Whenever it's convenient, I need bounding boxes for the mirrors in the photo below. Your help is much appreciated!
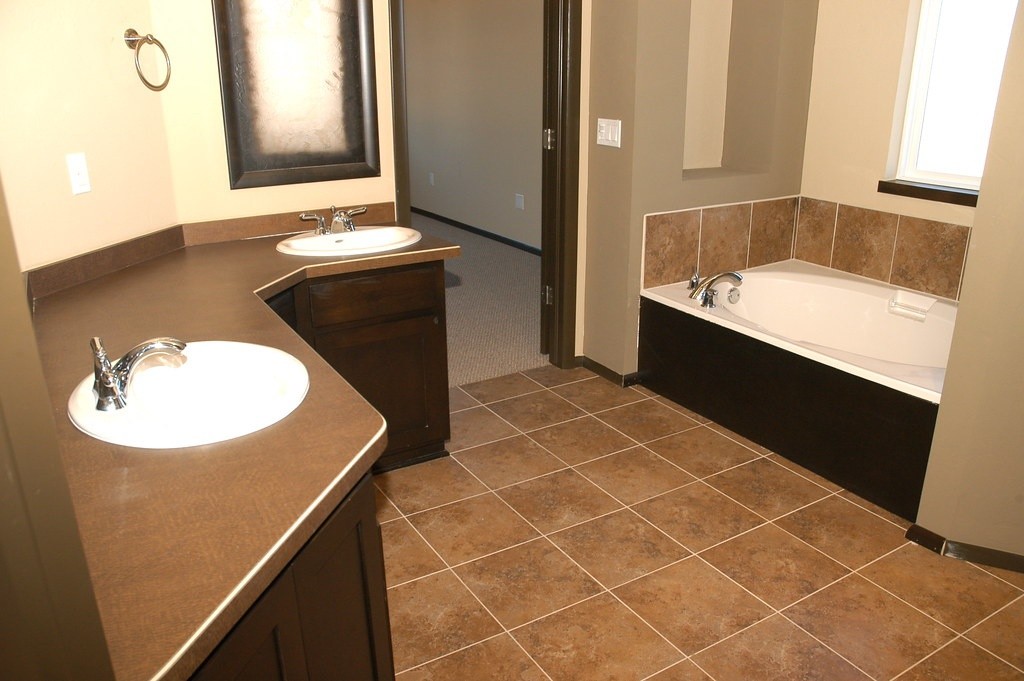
[211,0,382,192]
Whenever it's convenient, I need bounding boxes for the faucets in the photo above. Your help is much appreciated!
[112,334,187,385]
[689,268,743,300]
[330,210,355,233]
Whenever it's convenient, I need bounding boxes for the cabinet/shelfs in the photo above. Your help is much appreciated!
[177,462,397,681]
[267,259,450,476]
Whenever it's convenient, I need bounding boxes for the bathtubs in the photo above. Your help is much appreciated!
[636,257,959,530]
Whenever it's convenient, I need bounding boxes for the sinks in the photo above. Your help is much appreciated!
[275,222,423,257]
[67,335,312,451]
